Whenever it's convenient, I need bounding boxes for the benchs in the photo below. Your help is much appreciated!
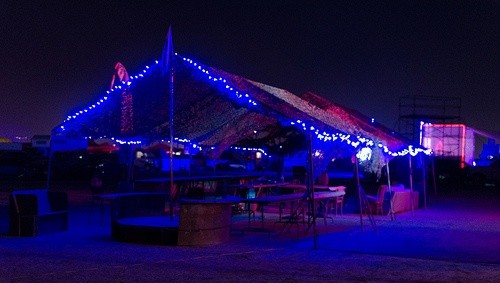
[94,171,421,240]
[9,188,70,240]
